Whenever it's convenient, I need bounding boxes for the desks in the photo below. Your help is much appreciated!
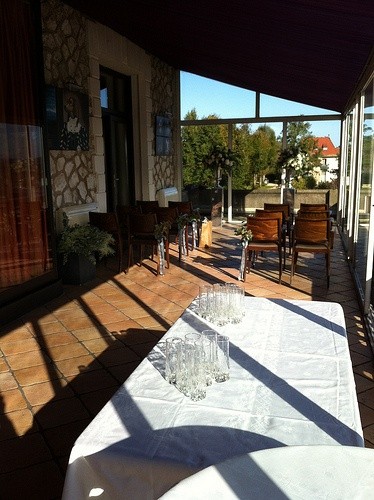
[62,293,365,500]
[158,445,374,500]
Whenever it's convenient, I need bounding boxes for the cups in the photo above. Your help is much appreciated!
[165,330,231,402]
[199,282,246,326]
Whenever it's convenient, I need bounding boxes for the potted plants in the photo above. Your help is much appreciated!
[54,213,116,285]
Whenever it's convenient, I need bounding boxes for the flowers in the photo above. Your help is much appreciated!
[278,145,307,171]
[202,145,234,171]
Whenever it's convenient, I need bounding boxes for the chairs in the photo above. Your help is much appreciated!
[241,203,334,289]
[89,200,202,277]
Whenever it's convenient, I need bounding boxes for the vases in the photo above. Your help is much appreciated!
[214,163,222,188]
[285,167,293,188]
